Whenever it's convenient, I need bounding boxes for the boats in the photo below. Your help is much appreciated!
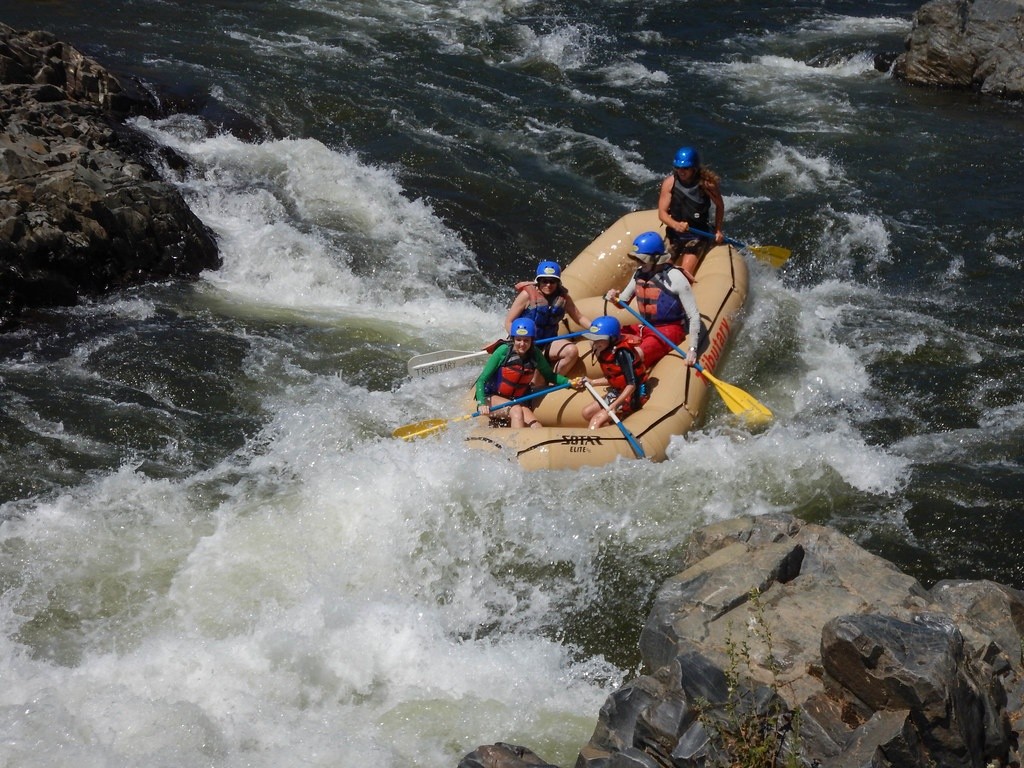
[460,209,751,469]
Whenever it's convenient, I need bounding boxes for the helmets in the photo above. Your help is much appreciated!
[633,231,665,255]
[535,261,561,278]
[672,146,698,167]
[590,315,620,337]
[510,317,536,336]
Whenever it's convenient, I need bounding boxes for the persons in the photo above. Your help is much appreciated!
[580,316,649,430]
[604,231,701,369]
[504,261,594,388]
[658,147,725,283]
[468,318,581,429]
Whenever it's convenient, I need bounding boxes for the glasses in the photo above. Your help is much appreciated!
[537,278,557,284]
[674,167,692,170]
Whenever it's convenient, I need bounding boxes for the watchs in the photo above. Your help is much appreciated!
[688,346,696,352]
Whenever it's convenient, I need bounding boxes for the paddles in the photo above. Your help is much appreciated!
[686,225,792,269]
[405,328,590,378]
[389,381,576,441]
[583,380,648,461]
[611,294,775,426]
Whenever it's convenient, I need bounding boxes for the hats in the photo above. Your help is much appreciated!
[533,275,562,288]
[581,333,616,342]
[627,252,671,265]
